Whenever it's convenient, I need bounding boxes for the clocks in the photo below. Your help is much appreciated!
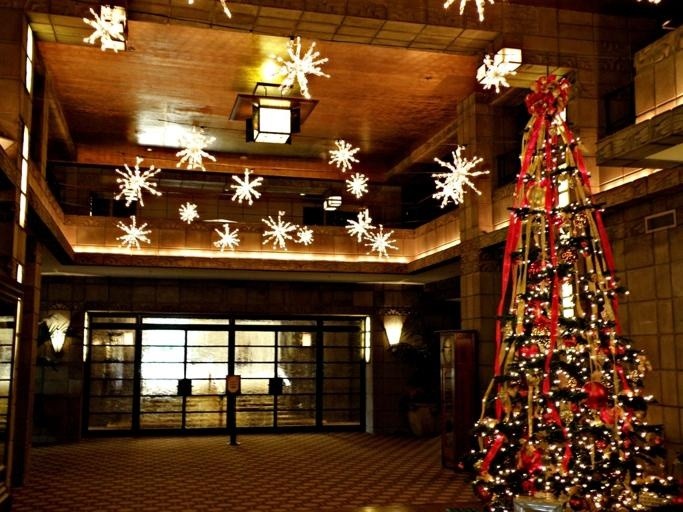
[433,330,476,470]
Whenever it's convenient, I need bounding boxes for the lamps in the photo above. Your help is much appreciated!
[477,48,522,84]
[322,189,342,212]
[38,304,80,353]
[378,307,412,352]
[228,94,320,146]
